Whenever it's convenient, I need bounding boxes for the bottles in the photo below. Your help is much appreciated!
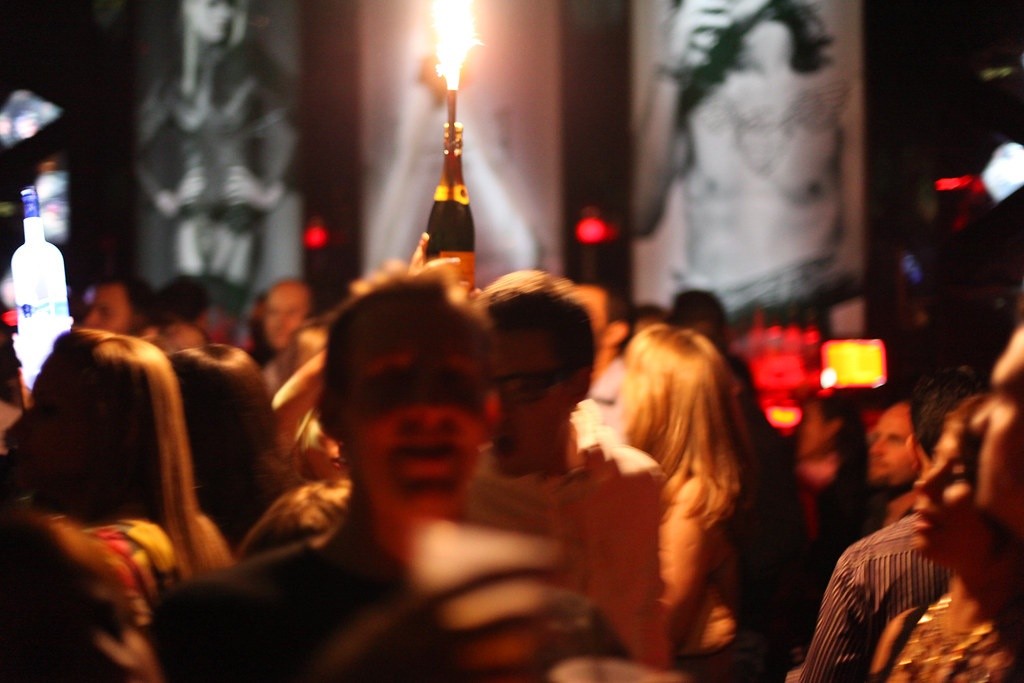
[427,121,476,290]
[15,187,71,409]
[747,303,826,388]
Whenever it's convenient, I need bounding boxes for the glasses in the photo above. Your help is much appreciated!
[336,366,488,415]
[484,364,586,405]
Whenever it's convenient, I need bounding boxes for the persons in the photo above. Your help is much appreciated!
[0,234,1024,683]
[134,0,298,283]
[633,0,849,307]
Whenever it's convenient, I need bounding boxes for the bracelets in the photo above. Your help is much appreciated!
[651,60,695,88]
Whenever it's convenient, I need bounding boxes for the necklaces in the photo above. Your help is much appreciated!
[897,594,1004,666]
